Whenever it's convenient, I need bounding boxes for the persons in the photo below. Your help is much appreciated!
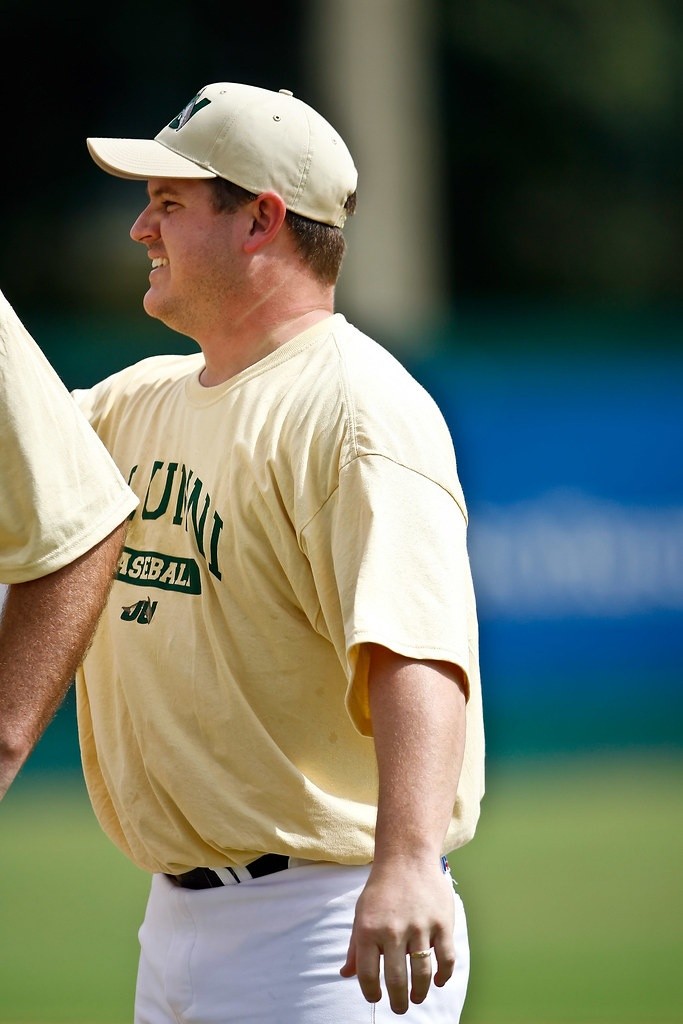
[0,291,141,803]
[69,82,486,1023]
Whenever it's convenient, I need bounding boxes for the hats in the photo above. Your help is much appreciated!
[86,82,359,229]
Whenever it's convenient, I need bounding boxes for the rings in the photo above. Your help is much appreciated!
[410,949,431,959]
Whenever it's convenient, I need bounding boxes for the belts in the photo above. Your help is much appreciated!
[164,852,289,891]
